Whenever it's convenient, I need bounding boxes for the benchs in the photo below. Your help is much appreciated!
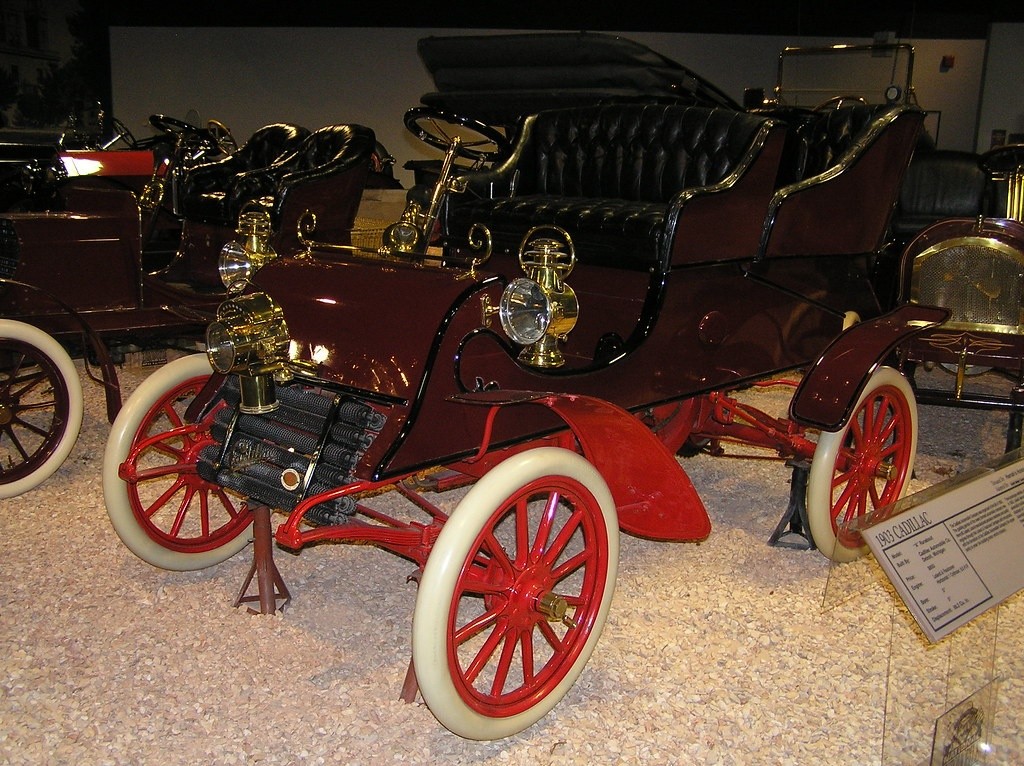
[445,103,786,270]
[746,102,928,258]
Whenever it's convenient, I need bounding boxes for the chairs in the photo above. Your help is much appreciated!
[183,124,312,226]
[895,215,1024,454]
[228,123,377,232]
